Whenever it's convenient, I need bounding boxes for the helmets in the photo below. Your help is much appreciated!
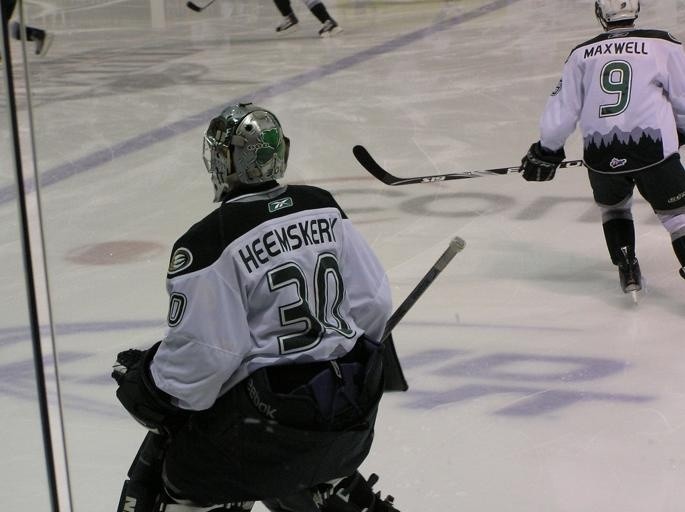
[202,103,290,202]
[595,0,640,23]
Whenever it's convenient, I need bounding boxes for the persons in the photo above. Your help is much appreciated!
[522,3,685,301]
[272,1,344,39]
[9,22,53,58]
[106,102,410,511]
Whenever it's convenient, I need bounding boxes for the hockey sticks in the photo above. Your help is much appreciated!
[187,1,215,12]
[353,145,586,186]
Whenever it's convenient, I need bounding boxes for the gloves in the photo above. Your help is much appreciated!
[518,141,565,181]
[111,341,190,436]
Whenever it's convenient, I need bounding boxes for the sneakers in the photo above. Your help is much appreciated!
[31,28,44,55]
[318,18,338,33]
[619,257,641,292]
[276,13,298,32]
